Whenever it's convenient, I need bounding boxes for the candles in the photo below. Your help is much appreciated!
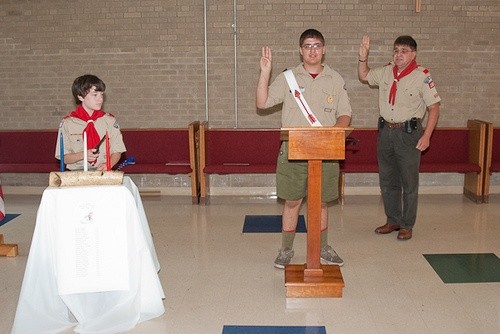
[60,130,65,172]
[83,130,88,172]
[105,130,111,171]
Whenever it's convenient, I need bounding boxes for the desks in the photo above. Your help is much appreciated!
[12,176,166,333]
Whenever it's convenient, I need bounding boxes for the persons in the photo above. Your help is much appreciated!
[55,73,127,172]
[358,35,441,239]
[256,29,352,269]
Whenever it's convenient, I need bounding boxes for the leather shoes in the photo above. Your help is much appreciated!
[376,224,400,233]
[397,229,412,239]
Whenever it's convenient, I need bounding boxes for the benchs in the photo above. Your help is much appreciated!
[0,121,500,204]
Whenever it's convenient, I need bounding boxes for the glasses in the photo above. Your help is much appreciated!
[392,50,412,54]
[302,43,322,50]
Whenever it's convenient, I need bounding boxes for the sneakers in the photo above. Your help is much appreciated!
[318,245,344,266]
[274,249,293,269]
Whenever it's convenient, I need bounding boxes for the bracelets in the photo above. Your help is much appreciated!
[359,59,367,62]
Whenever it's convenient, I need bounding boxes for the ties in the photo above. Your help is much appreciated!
[388,60,416,105]
[70,104,107,164]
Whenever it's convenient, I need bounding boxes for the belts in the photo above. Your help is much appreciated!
[386,121,407,128]
